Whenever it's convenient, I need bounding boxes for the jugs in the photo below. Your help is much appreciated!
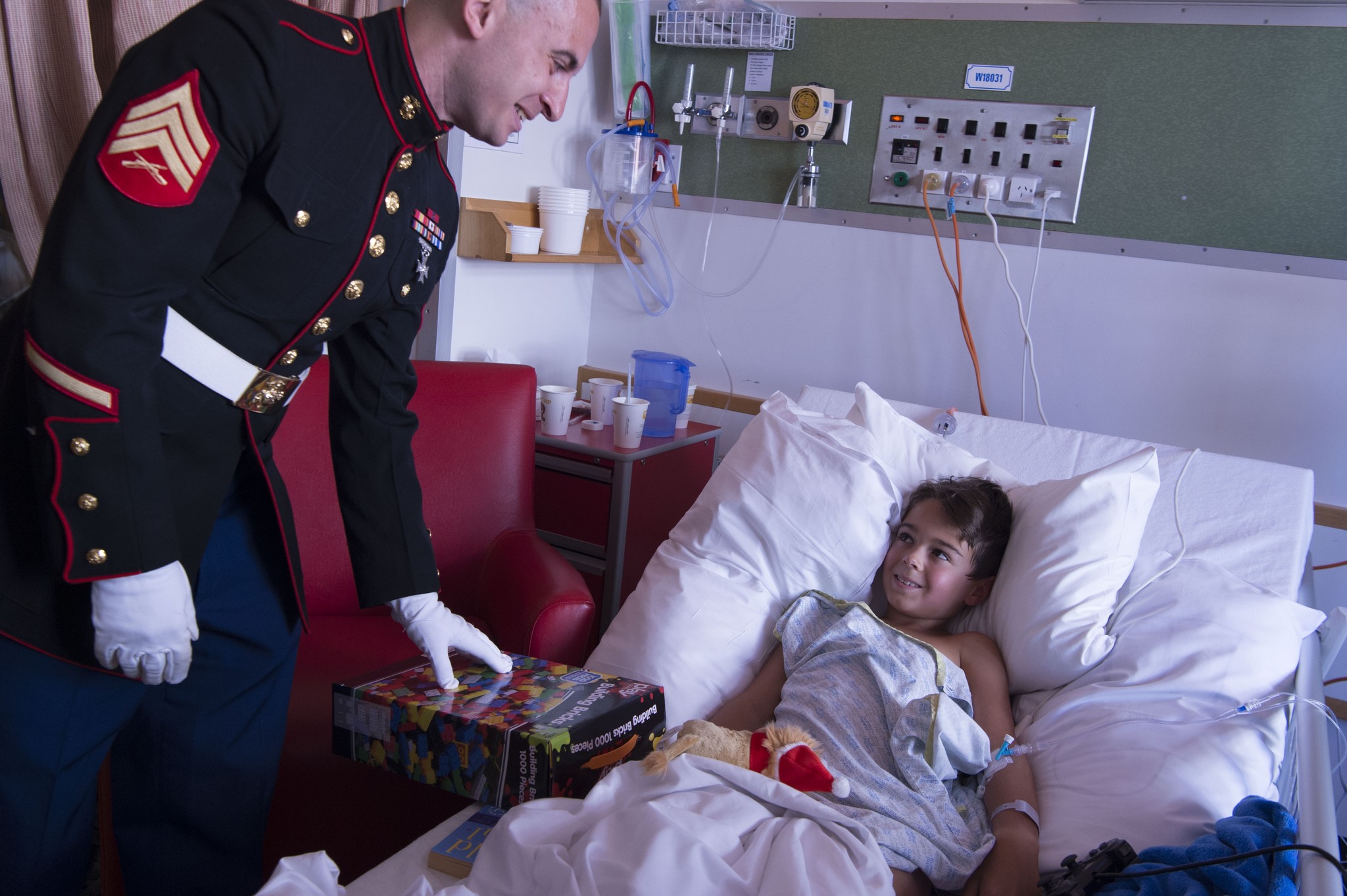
[630,349,697,437]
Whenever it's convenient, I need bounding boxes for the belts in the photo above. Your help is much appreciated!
[158,306,312,417]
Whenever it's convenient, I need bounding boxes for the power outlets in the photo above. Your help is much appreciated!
[654,145,682,193]
[920,168,948,195]
[977,174,1005,201]
[1007,177,1037,203]
[949,170,977,198]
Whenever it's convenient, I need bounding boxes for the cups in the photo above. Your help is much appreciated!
[668,381,697,429]
[611,397,650,448]
[508,226,544,254]
[538,186,590,254]
[588,378,624,425]
[539,386,577,436]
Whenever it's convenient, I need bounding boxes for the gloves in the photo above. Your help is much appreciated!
[384,592,513,689]
[90,561,199,686]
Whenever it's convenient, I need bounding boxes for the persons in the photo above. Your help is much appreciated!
[0,0,602,896]
[712,474,1042,896]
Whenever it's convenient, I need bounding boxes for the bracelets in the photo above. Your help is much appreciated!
[989,799,1040,831]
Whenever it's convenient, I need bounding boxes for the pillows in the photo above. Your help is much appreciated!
[844,381,1160,689]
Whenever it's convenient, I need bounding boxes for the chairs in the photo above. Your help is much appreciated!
[258,356,596,886]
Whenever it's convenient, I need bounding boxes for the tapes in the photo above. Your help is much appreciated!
[581,419,604,430]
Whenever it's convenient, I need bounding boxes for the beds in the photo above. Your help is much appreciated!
[254,381,1344,896]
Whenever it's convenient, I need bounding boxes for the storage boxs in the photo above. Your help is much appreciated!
[331,646,667,809]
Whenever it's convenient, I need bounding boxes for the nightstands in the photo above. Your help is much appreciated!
[534,408,721,667]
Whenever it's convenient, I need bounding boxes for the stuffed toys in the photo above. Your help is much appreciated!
[642,718,852,799]
